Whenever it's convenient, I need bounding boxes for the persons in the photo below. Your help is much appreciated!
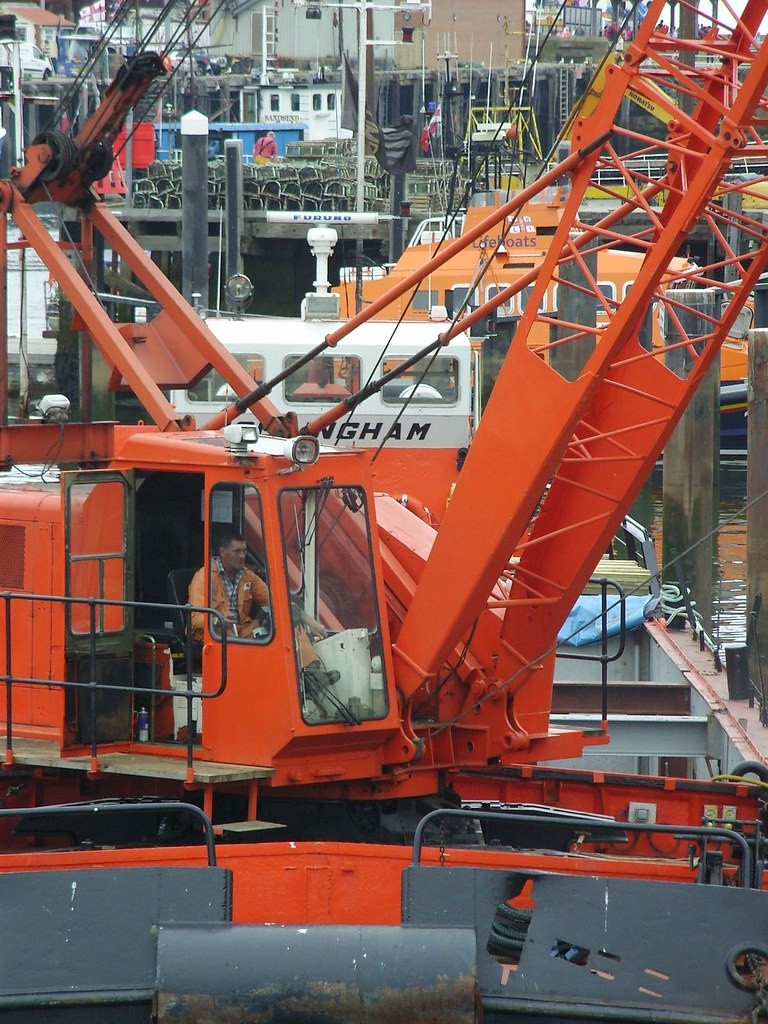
[185,528,341,696]
[43,40,51,61]
[314,364,329,388]
[575,19,713,43]
[252,131,279,167]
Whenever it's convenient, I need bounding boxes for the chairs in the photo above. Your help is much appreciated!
[166,567,204,657]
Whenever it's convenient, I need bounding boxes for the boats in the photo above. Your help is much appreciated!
[168,191,756,476]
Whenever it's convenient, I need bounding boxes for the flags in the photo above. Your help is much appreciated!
[421,104,441,153]
[154,100,162,124]
[159,52,172,75]
[79,0,106,23]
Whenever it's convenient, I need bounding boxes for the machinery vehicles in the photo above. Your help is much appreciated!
[0,0,768,1024]
